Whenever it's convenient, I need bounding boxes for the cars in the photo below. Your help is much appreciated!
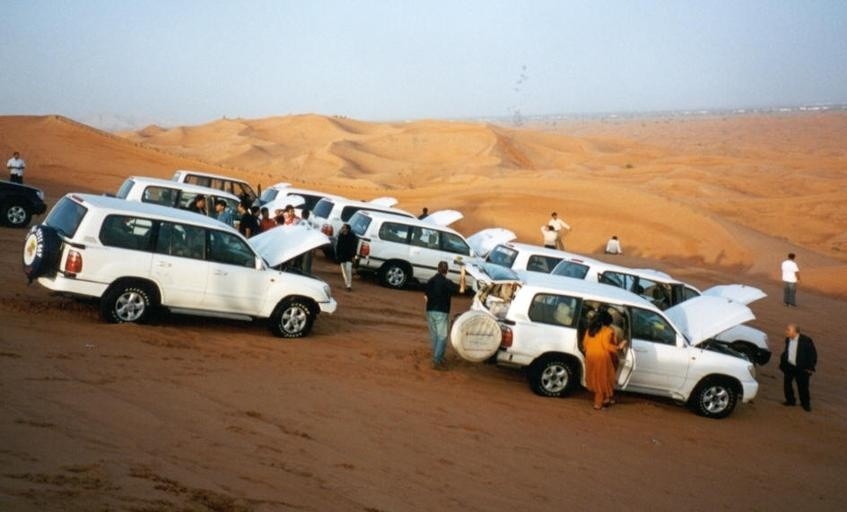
[0,176,48,227]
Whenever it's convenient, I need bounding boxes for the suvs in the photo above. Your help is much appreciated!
[465,235,671,308]
[18,190,341,341]
[447,257,764,422]
[547,254,772,369]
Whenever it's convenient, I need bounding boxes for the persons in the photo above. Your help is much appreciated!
[5,150,26,183]
[580,310,629,411]
[539,224,558,248]
[779,253,800,306]
[335,224,357,292]
[190,194,313,276]
[423,259,465,370]
[547,211,570,249]
[604,235,622,255]
[776,321,816,413]
[416,207,428,220]
